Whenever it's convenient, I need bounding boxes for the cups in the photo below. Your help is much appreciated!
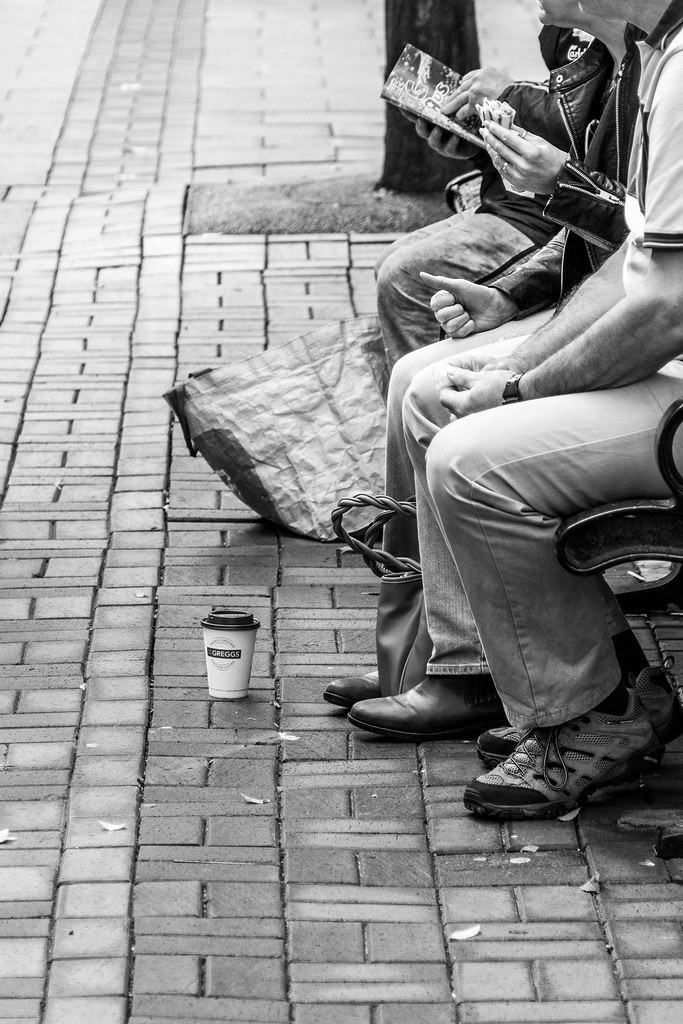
[201,610,261,698]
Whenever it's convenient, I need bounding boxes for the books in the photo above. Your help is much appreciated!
[379,42,486,150]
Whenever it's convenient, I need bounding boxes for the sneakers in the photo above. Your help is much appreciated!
[476,653,683,768]
[463,673,666,820]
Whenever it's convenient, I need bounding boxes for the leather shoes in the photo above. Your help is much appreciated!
[347,674,508,742]
[323,670,382,707]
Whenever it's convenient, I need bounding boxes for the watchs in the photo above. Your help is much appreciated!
[502,372,527,404]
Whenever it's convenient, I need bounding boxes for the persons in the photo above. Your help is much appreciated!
[323,0,683,821]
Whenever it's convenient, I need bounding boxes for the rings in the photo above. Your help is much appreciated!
[503,161,509,175]
[521,130,528,139]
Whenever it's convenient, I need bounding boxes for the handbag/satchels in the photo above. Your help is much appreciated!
[161,313,391,543]
[330,492,435,699]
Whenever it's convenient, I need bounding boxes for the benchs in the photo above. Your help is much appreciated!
[443,166,683,612]
